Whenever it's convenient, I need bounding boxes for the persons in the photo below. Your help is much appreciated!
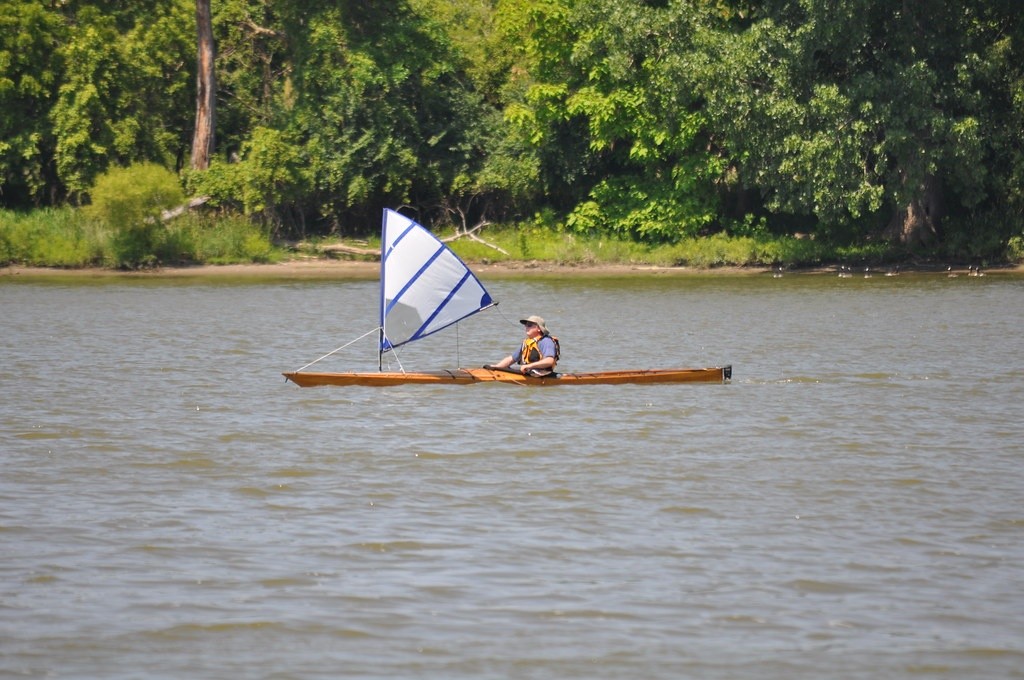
[491,316,557,376]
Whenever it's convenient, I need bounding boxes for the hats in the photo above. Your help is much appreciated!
[519,316,550,336]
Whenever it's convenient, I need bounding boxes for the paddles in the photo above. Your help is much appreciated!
[482,365,558,378]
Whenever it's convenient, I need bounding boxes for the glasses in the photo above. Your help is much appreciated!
[525,323,537,327]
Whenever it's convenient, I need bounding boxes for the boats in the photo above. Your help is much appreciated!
[285,365,732,389]
